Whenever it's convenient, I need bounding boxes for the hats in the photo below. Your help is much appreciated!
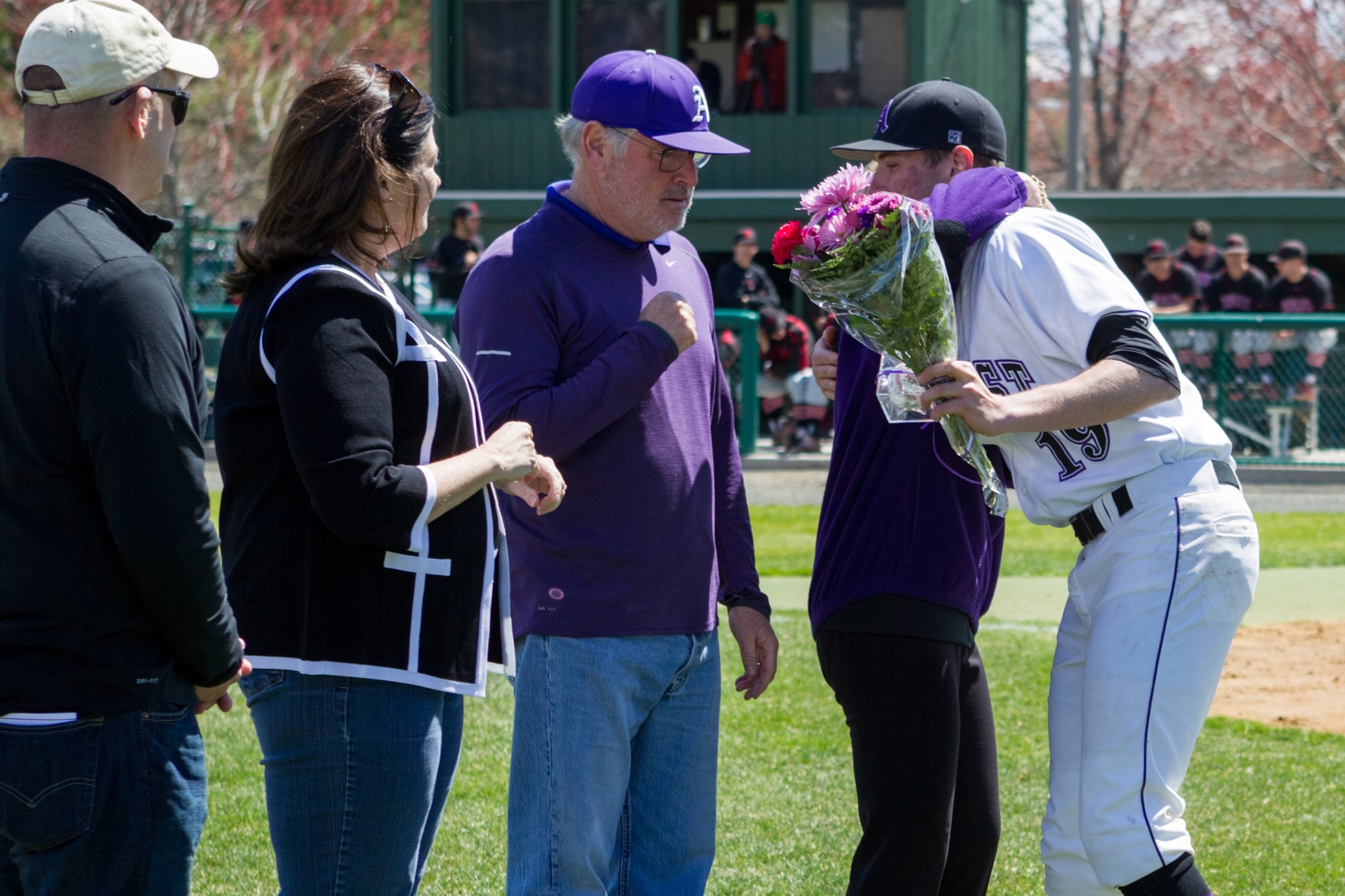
[1220,232,1249,255]
[759,308,787,336]
[450,200,490,218]
[1266,238,1308,263]
[571,49,751,155]
[1188,218,1214,242]
[1144,237,1169,261]
[829,77,1007,163]
[15,0,219,108]
[733,228,759,247]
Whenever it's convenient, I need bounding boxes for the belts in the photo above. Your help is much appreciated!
[1069,460,1239,546]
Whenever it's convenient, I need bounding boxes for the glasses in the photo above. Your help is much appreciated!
[110,85,190,126]
[376,60,423,162]
[607,126,712,172]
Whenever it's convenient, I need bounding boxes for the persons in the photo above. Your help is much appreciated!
[208,58,571,896]
[458,48,782,896]
[0,0,249,896]
[797,75,1260,896]
[194,10,1338,462]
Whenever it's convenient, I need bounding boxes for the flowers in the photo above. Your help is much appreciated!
[771,163,1013,522]
